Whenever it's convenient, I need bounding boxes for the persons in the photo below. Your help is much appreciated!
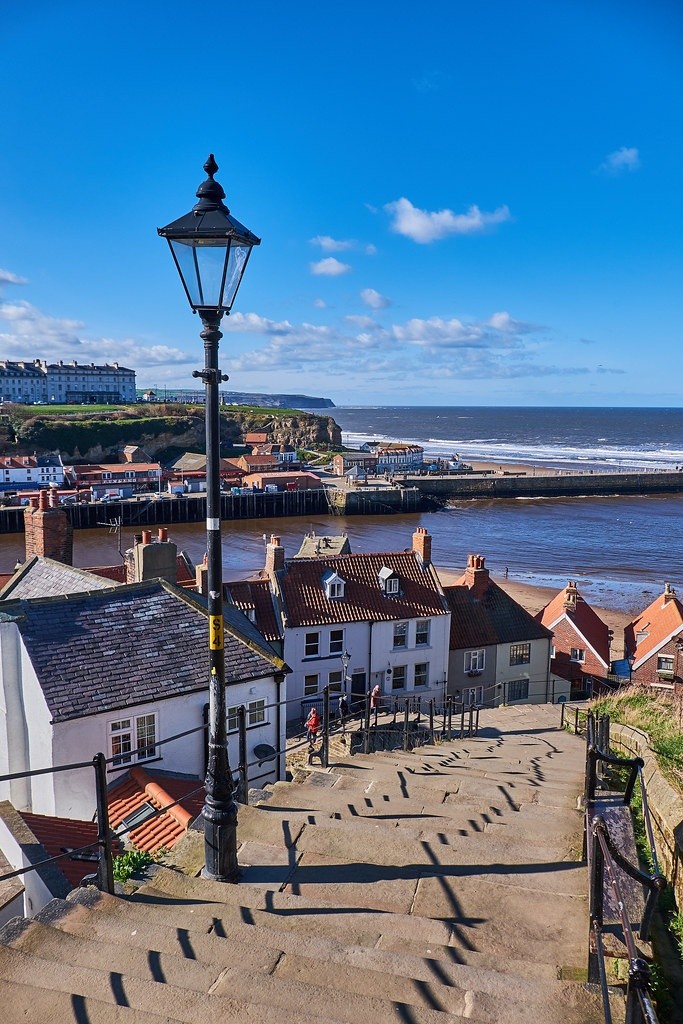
[370,685,380,713]
[304,708,319,743]
[338,696,348,725]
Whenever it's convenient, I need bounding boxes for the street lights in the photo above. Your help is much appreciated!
[156,149,265,891]
